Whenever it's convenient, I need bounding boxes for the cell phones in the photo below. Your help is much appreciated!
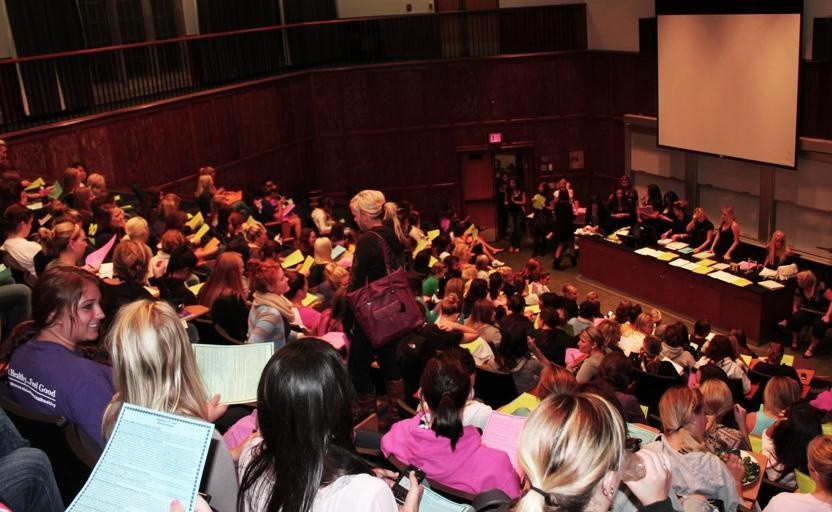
[391,464,426,505]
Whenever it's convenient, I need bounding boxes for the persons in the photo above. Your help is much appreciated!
[1,158,832,511]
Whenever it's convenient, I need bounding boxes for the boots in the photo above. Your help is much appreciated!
[350,392,378,426]
[377,377,407,433]
[570,254,577,266]
[552,257,566,272]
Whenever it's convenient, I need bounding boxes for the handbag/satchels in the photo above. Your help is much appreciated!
[343,266,427,351]
